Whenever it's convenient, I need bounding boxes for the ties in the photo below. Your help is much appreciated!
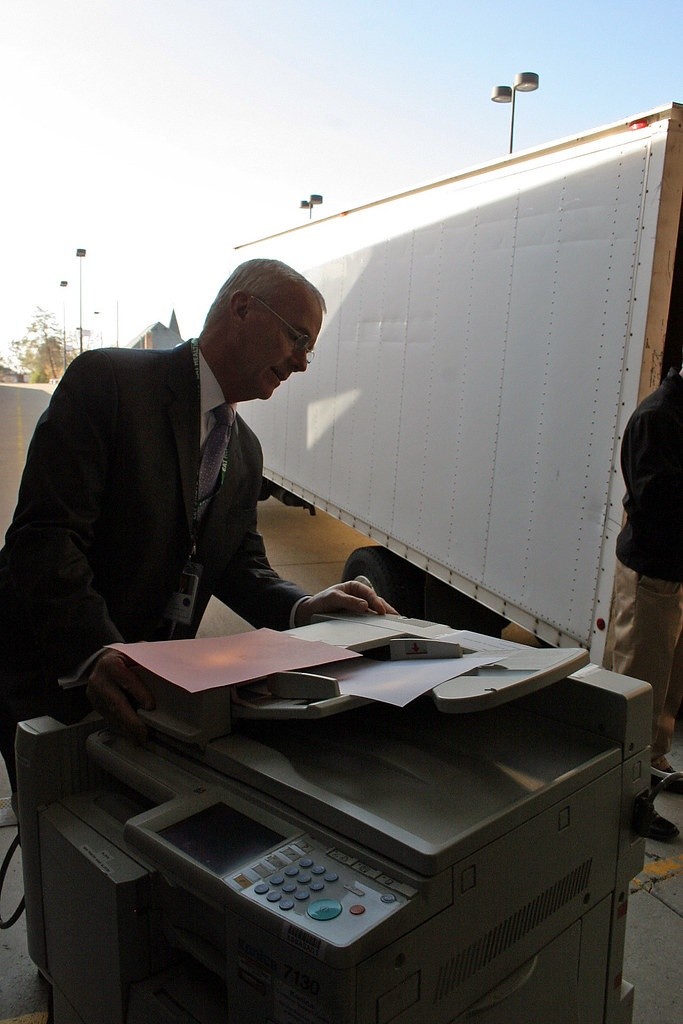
[196,403,235,538]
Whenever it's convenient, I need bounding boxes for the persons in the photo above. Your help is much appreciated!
[611,367,683,840]
[0,260,397,834]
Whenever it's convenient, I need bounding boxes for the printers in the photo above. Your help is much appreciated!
[14,604,678,1024]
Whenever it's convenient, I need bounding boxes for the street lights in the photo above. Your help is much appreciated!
[490,70,539,157]
[94,310,104,348]
[76,246,87,354]
[299,193,324,221]
[58,279,69,374]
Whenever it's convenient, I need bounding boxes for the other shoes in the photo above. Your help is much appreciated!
[651,766,683,794]
[645,809,679,842]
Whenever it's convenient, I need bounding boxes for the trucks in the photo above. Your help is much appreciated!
[198,105,682,706]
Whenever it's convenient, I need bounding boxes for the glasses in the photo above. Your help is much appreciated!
[251,294,315,364]
